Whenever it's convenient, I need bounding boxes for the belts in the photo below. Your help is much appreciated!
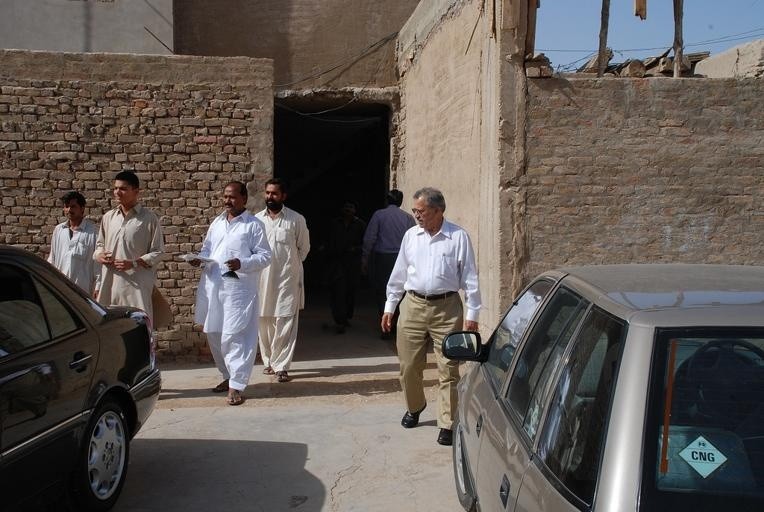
[406,290,456,302]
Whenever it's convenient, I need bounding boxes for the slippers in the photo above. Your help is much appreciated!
[211,379,229,393]
[262,366,274,375]
[225,388,243,406]
[276,370,289,382]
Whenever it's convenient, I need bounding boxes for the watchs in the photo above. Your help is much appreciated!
[131,259,138,271]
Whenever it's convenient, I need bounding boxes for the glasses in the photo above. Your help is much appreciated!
[410,208,425,215]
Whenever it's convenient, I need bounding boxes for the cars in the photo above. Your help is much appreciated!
[441,265,763,511]
[1,237,160,512]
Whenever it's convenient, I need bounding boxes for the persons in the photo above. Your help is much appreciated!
[93,171,164,372]
[187,182,272,405]
[362,189,415,339]
[46,191,103,301]
[316,200,366,334]
[255,179,311,381]
[381,186,481,446]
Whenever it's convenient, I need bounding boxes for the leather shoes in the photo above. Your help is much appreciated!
[436,427,453,446]
[400,402,427,428]
